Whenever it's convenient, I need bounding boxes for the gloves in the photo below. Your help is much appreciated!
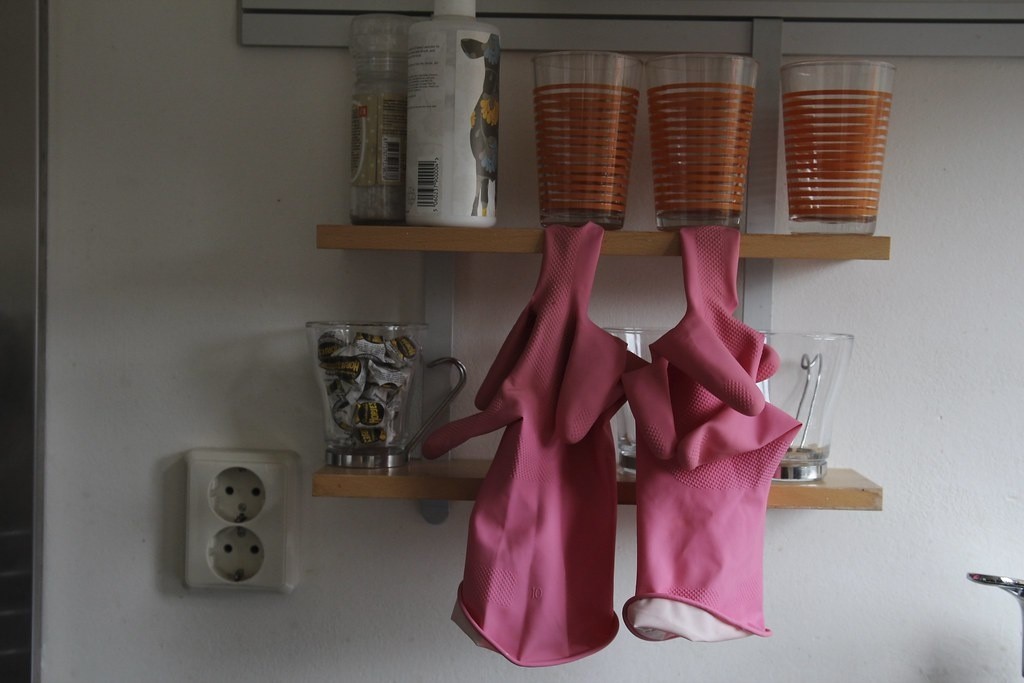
[421,220,803,666]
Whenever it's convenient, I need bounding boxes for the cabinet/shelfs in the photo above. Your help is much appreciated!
[308,222,894,514]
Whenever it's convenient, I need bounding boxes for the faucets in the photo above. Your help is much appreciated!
[967,573,1024,677]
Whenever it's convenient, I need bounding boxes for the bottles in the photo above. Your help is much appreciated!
[406,0,501,228]
[342,14,417,225]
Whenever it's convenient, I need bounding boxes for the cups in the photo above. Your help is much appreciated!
[530,51,644,230]
[645,53,760,232]
[780,60,897,236]
[304,320,469,469]
[759,331,856,483]
[603,327,672,478]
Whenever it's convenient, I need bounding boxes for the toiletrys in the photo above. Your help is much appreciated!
[407,1,500,225]
[349,17,415,221]
